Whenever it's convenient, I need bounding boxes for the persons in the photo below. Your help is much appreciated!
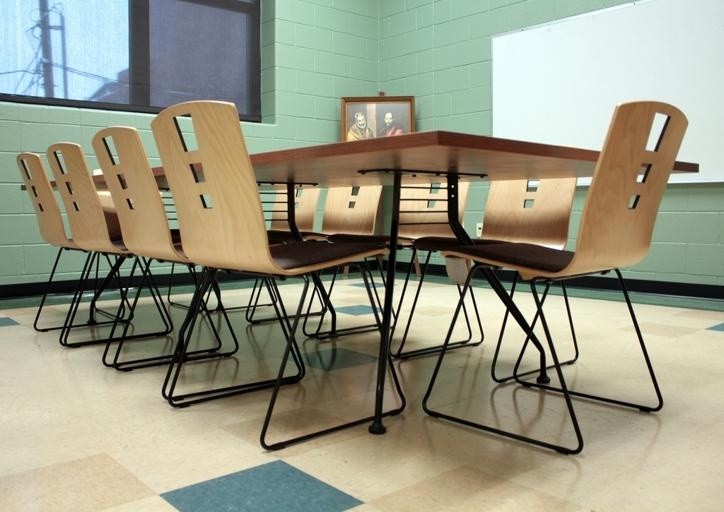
[347,111,373,142]
[375,112,404,138]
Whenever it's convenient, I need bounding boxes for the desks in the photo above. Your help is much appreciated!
[20,130,700,435]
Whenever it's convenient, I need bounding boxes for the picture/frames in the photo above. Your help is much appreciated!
[339,96,416,144]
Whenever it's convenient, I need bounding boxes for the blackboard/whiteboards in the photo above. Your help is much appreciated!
[492,1,724,182]
